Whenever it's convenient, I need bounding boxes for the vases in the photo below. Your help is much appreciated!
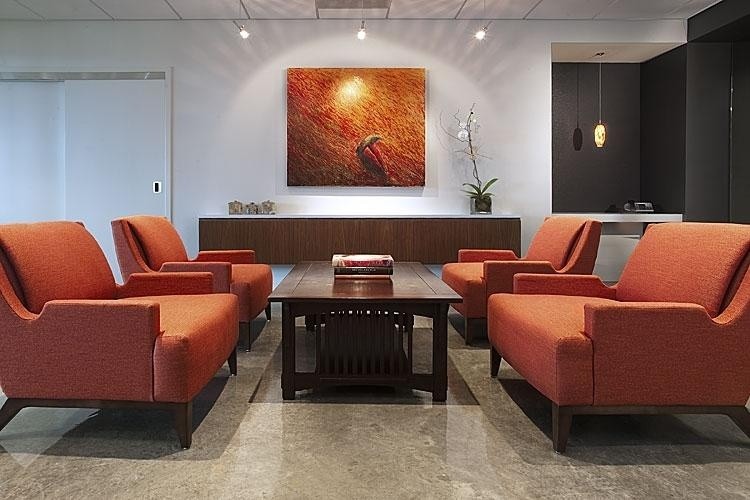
[470,199,493,215]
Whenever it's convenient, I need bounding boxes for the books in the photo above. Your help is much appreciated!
[333,274,391,279]
[331,252,395,268]
[333,267,394,276]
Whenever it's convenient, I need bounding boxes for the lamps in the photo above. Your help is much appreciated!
[593,51,607,148]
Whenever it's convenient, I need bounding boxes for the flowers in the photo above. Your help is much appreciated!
[439,97,500,199]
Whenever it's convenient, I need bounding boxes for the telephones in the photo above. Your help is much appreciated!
[624,200,654,213]
